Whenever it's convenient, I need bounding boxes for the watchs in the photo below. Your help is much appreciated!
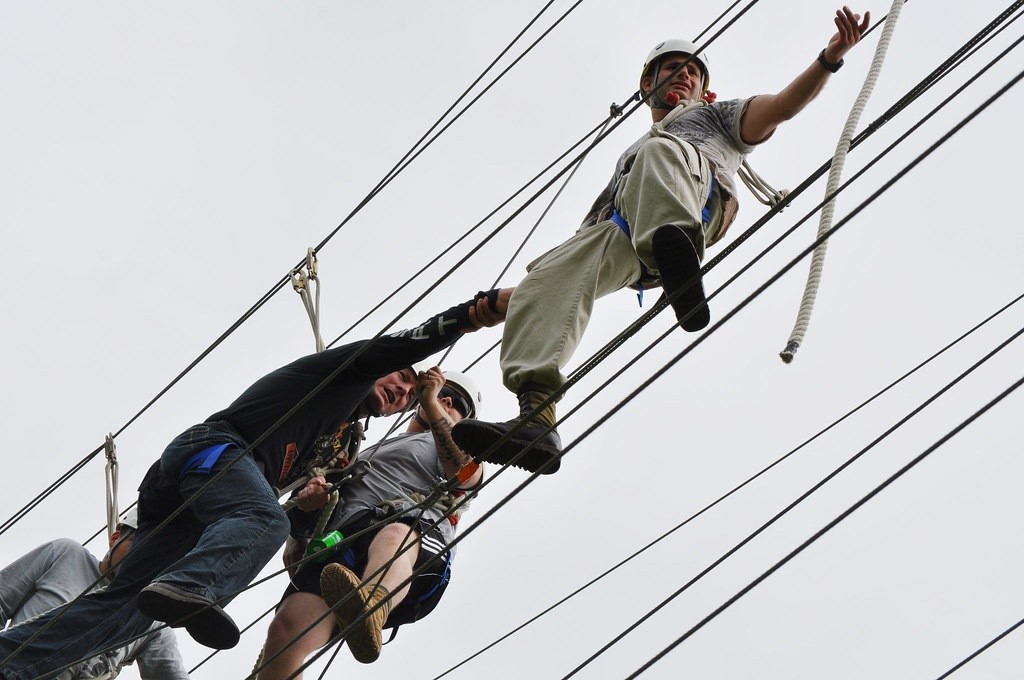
[818,48,844,73]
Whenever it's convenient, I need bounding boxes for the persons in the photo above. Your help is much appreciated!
[0,287,519,680]
[0,507,190,680]
[451,6,871,475]
[256,366,482,680]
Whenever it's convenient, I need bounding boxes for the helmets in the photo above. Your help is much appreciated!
[639,40,711,107]
[116,507,137,530]
[410,357,433,378]
[443,371,482,420]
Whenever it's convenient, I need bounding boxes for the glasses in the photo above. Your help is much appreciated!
[438,384,470,417]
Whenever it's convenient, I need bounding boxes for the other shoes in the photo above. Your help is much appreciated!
[136,583,239,649]
[653,225,710,332]
[451,417,561,475]
[320,563,392,663]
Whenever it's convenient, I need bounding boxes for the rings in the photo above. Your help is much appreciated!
[428,374,431,379]
